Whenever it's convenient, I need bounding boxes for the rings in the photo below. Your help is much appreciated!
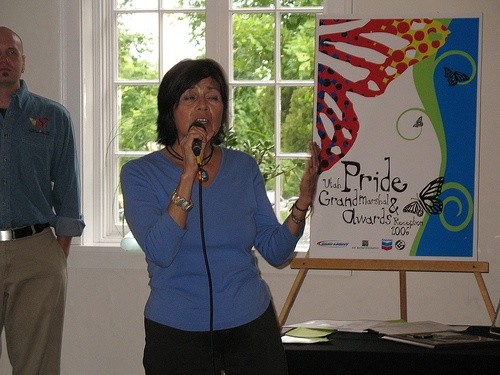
[309,166,313,168]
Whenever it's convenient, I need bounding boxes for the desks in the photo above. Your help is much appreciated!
[278,320,500,375]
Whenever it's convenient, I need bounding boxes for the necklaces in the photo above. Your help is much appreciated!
[166,145,214,182]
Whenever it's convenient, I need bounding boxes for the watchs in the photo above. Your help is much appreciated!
[170,191,194,213]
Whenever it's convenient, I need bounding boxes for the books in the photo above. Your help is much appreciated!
[383,332,500,348]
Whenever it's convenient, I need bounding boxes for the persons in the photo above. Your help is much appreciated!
[414,334,490,341]
[0,25,86,375]
[120,58,322,375]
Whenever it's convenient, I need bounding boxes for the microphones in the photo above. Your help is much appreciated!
[187,120,206,157]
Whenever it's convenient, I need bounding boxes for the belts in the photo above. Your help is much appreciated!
[0,222,49,242]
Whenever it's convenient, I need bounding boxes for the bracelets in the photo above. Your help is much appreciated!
[289,199,308,211]
[291,210,305,224]
